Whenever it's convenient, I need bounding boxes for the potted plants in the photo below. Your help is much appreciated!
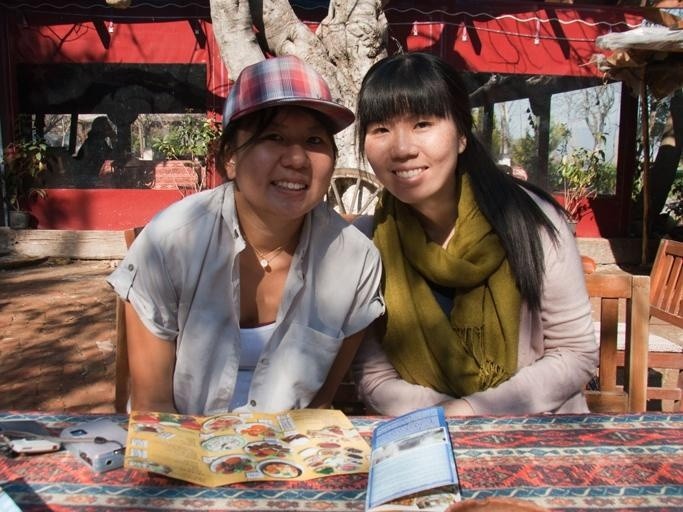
[0,134,47,229]
[558,131,616,236]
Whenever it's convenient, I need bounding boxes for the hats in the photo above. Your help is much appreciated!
[222,54,355,134]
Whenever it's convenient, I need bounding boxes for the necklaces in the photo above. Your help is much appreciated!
[239,233,293,275]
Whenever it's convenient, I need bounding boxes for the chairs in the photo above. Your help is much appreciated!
[115,295,131,412]
[585,273,648,412]
[124,228,142,250]
[592,237,683,411]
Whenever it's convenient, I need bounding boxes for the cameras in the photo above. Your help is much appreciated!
[62,417,129,473]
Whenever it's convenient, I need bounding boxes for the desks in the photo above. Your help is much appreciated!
[2,409,682,511]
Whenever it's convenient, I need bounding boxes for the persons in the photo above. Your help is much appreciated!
[106,55,388,416]
[336,49,602,419]
[50,116,112,187]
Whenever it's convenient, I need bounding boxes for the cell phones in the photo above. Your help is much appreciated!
[0,414,62,455]
